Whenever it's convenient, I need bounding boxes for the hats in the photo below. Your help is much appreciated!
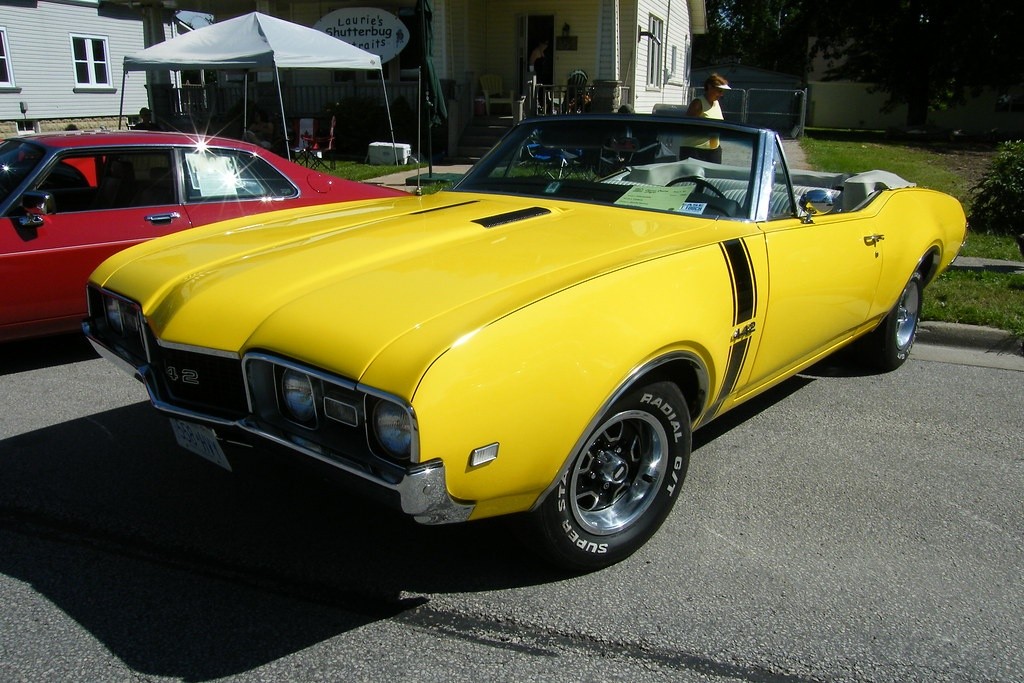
[716,84,732,90]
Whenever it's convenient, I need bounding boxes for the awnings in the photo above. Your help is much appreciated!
[119,11,399,167]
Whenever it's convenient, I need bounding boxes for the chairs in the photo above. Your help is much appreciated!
[1,69,841,220]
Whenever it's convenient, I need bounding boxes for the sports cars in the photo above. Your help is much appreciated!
[80,116,969,571]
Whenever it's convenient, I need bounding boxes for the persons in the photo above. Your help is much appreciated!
[528,38,551,106]
[130,108,162,131]
[245,110,273,151]
[680,73,731,164]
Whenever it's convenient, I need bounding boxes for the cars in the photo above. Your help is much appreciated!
[0,133,418,347]
